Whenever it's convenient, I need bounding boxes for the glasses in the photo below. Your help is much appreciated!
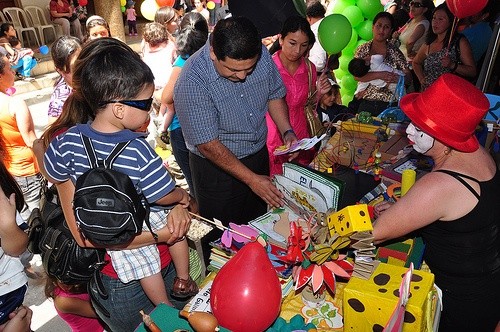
[124,98,153,113]
[409,1,424,9]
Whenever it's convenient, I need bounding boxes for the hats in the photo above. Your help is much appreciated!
[124,0,135,8]
[399,71,491,152]
[316,79,341,91]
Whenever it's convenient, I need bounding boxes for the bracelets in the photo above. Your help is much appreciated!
[281,130,293,139]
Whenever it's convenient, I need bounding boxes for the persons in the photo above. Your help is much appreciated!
[305,0,327,78]
[0,0,198,332]
[138,0,221,194]
[174,16,301,277]
[348,0,500,117]
[315,78,349,134]
[371,73,500,332]
[264,17,331,178]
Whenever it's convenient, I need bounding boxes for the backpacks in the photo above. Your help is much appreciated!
[70,131,144,248]
[25,202,64,254]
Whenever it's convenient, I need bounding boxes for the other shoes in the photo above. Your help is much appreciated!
[134,33,139,36]
[128,32,132,36]
[174,274,199,294]
[23,77,35,82]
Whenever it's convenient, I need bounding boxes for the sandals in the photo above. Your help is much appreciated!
[155,127,169,145]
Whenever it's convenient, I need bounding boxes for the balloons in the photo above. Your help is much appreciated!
[210,236,282,332]
[318,0,384,109]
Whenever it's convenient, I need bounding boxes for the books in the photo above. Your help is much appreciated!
[209,239,294,297]
[249,163,340,245]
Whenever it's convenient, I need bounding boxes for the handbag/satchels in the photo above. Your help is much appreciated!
[38,219,106,290]
[303,58,328,143]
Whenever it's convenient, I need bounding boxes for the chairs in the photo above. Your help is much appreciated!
[0,6,40,49]
[24,7,57,46]
